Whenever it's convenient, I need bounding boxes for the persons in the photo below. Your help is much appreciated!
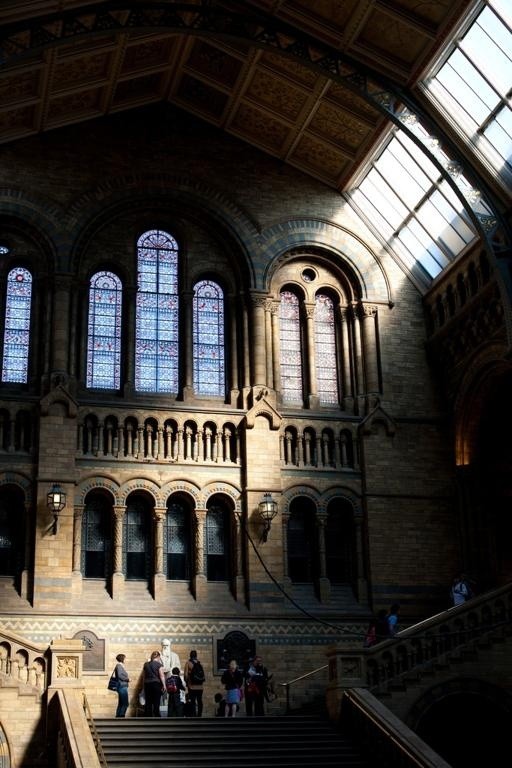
[363,620,380,677]
[158,638,182,679]
[140,650,271,718]
[450,576,469,605]
[373,607,390,642]
[387,602,401,634]
[114,653,132,716]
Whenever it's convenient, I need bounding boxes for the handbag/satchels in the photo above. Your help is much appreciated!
[108,676,120,691]
[139,689,146,705]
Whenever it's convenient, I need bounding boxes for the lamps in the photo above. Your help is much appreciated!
[46,484,67,535]
[259,492,278,542]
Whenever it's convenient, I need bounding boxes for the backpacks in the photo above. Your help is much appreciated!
[187,659,205,686]
[166,675,179,694]
